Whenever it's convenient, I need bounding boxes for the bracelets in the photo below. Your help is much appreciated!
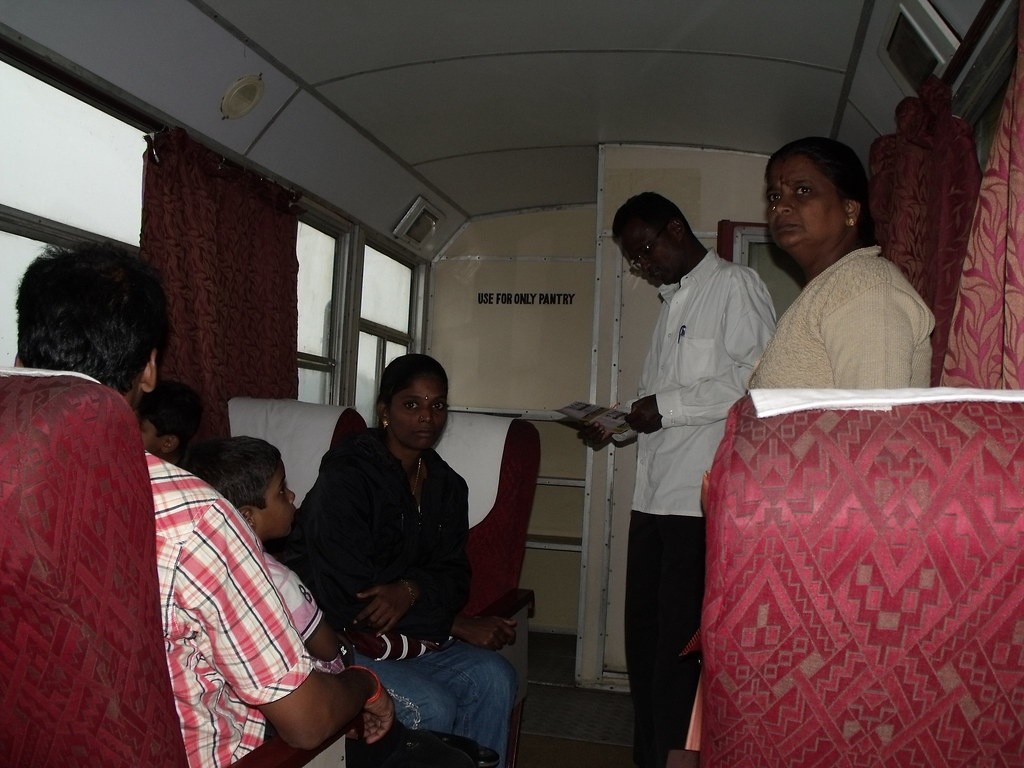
[399,578,416,608]
[347,664,382,706]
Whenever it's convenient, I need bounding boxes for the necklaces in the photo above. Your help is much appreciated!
[412,456,423,496]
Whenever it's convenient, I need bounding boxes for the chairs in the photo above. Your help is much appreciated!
[0,366,368,768]
[666,389,1024,768]
[377,412,542,768]
[226,397,368,512]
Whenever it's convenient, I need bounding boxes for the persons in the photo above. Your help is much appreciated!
[747,136,936,390]
[14,239,395,768]
[578,191,778,768]
[277,353,519,768]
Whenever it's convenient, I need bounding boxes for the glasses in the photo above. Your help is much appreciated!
[629,224,668,271]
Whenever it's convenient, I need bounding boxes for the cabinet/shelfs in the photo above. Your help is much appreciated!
[447,407,586,768]
